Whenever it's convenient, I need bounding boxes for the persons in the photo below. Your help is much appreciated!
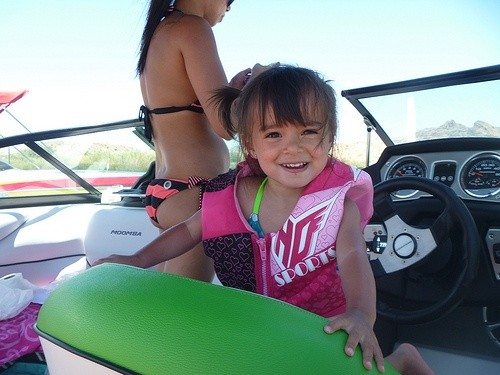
[134,0,275,287]
[87,63,438,375]
